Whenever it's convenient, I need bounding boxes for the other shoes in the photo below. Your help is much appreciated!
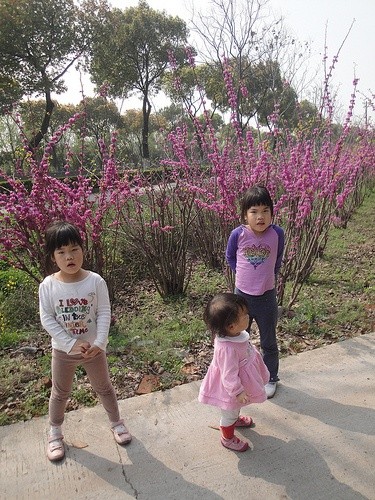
[109,418,131,445]
[233,415,252,426]
[46,431,64,460]
[221,435,248,452]
[264,382,277,397]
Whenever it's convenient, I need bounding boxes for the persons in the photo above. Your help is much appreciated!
[36,219,133,462]
[195,291,271,453]
[224,183,286,400]
